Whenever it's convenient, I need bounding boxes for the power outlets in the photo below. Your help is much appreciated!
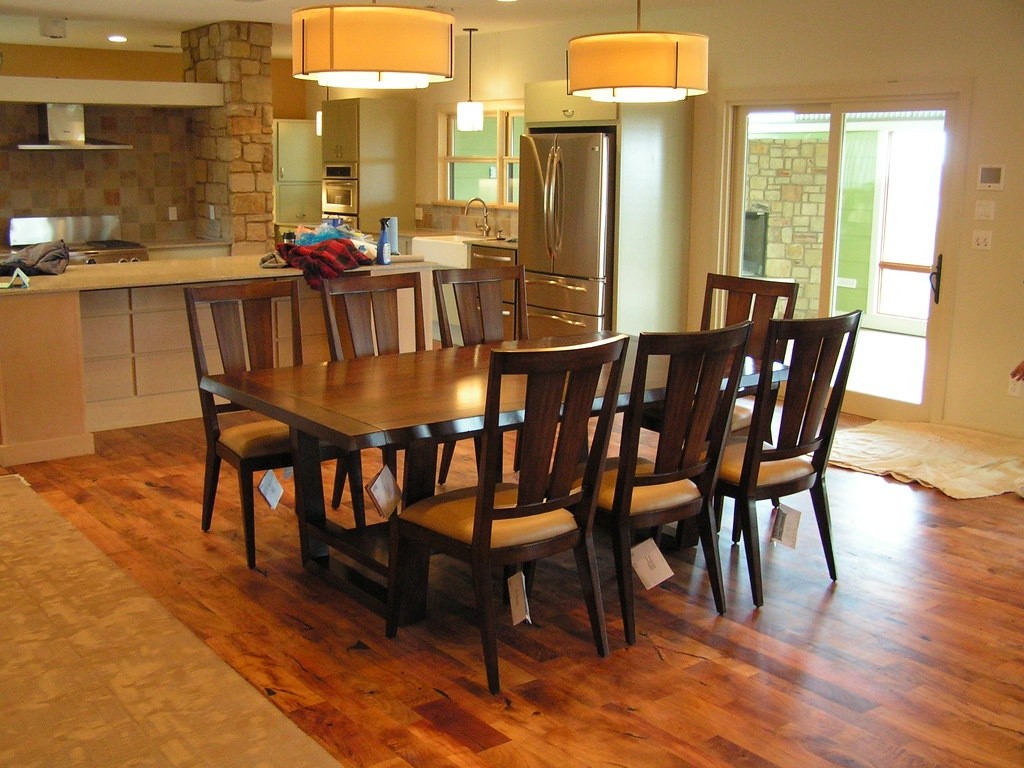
[971,229,992,251]
[415,208,422,220]
[168,207,178,220]
[209,205,216,220]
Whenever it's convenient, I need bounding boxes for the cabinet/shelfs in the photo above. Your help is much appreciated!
[322,97,417,231]
[464,238,520,340]
[273,117,323,226]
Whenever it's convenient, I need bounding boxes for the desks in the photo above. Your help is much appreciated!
[0,252,442,468]
[202,331,791,626]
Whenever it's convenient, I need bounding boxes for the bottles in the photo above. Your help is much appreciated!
[283,233,296,244]
[386,217,398,254]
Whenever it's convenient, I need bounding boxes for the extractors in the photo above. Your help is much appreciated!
[0,103,133,150]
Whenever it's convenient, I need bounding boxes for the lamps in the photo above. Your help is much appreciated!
[457,28,484,132]
[565,0,711,104]
[290,0,456,90]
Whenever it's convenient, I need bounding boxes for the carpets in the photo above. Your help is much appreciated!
[808,418,1024,501]
[0,471,348,768]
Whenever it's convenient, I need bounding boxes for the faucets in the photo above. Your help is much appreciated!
[464,197,491,236]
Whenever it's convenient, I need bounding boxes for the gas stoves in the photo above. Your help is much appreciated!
[8,214,149,265]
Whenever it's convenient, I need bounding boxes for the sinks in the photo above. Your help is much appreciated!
[411,235,482,270]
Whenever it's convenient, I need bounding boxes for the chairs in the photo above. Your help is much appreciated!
[321,272,428,541]
[545,319,756,645]
[432,265,532,483]
[698,309,863,607]
[384,332,632,697]
[182,279,367,569]
[641,271,802,536]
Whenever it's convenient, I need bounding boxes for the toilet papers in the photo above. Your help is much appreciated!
[387,217,398,254]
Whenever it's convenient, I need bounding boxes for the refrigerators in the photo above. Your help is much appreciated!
[519,132,616,337]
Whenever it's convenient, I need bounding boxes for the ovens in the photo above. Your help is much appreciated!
[322,162,358,215]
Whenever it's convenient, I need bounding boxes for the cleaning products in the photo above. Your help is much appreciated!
[376,218,391,265]
